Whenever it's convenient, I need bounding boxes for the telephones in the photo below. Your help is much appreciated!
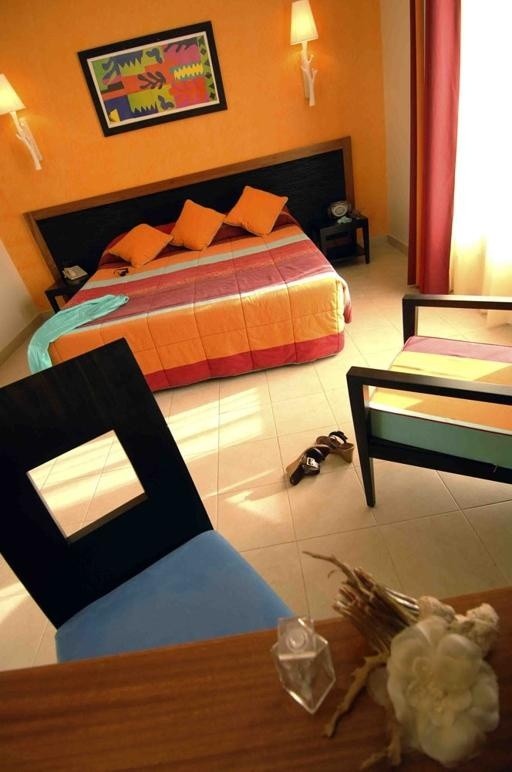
[62,265,88,281]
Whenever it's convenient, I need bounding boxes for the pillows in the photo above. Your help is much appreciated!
[26,194,352,393]
[224,186,290,239]
[169,199,227,254]
[109,222,175,269]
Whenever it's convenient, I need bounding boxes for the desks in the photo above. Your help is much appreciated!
[44,265,98,314]
[304,212,371,265]
[1,583,509,772]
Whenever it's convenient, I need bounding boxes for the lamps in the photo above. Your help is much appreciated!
[0,71,45,172]
[287,1,323,109]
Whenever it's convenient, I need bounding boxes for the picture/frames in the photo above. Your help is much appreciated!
[76,19,230,139]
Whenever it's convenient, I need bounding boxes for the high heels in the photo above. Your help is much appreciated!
[285,445,331,488]
[316,430,354,463]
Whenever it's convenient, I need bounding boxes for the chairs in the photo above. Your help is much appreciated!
[344,292,511,509]
[1,335,295,663]
